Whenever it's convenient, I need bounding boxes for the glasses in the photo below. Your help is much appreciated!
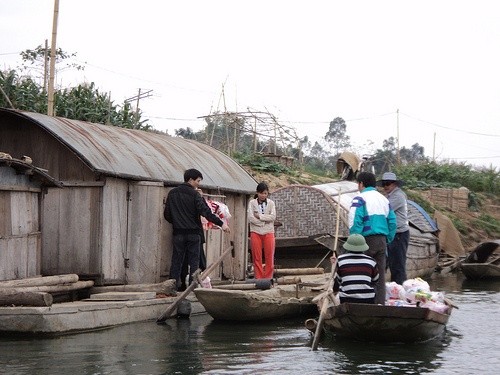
[381,180,396,187]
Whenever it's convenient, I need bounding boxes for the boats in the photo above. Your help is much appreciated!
[459,237,500,279]
[194,180,442,324]
[303,294,453,340]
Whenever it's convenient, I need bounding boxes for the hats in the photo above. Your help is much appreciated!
[343,234,369,252]
[376,173,404,187]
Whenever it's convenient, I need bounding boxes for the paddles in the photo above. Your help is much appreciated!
[311,261,338,350]
[157,240,236,323]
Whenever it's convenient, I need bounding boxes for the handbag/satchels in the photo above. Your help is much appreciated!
[385,276,447,313]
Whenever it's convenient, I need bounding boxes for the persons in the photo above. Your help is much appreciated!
[330,233,380,304]
[164,168,231,294]
[376,172,410,286]
[247,183,276,279]
[347,172,397,306]
